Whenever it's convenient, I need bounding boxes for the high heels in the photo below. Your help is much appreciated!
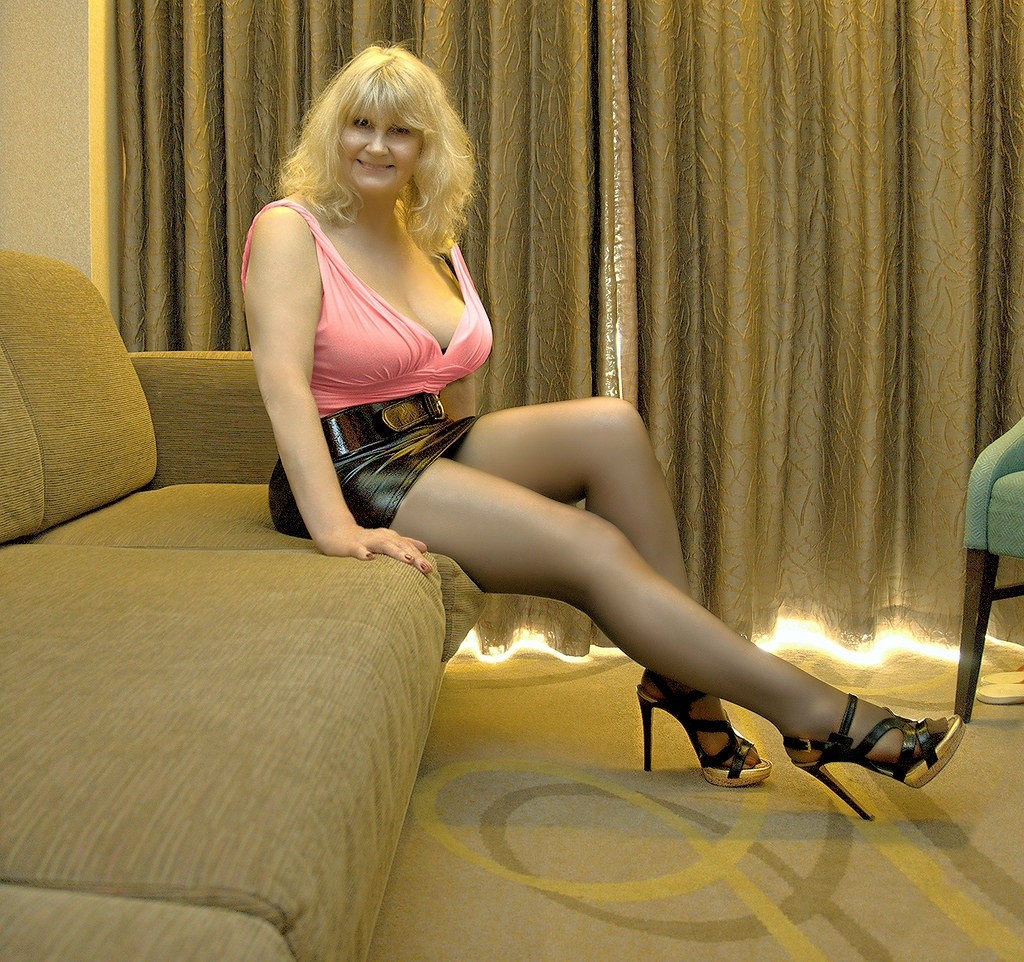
[636,667,773,788]
[780,691,967,824]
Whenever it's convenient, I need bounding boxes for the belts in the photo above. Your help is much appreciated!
[319,393,448,457]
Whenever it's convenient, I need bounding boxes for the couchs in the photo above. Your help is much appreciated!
[0,250,487,962]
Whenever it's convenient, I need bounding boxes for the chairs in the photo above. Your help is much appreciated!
[953,412,1024,723]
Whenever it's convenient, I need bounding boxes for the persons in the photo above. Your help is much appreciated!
[240,40,966,820]
